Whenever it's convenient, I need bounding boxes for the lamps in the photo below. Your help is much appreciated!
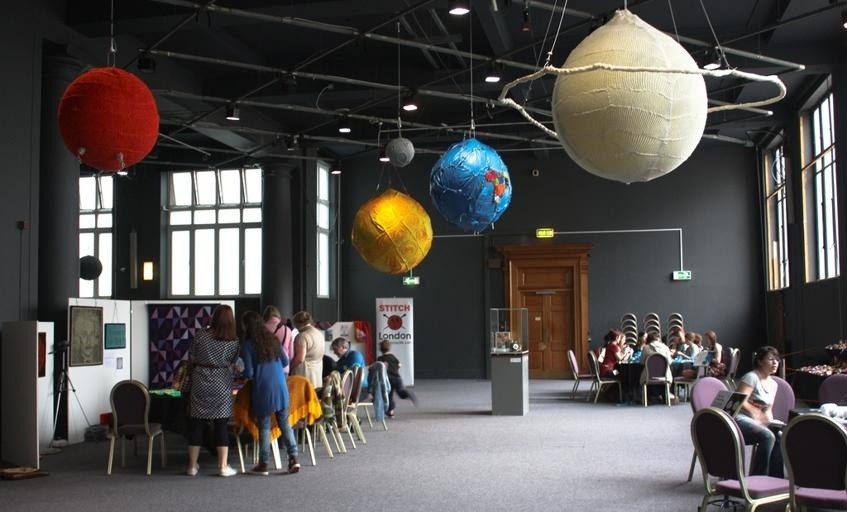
[698,41,720,70]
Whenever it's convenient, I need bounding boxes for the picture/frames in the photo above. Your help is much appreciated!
[105,323,126,349]
[70,305,103,366]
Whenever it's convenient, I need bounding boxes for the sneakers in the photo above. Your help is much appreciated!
[288,457,301,474]
[186,462,201,477]
[247,463,270,476]
[218,465,238,477]
[385,410,395,419]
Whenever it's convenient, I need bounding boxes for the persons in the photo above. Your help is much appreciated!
[378,340,418,418]
[289,311,326,443]
[598,328,726,404]
[733,345,785,479]
[331,337,369,432]
[181,305,240,476]
[262,306,294,373]
[239,311,301,476]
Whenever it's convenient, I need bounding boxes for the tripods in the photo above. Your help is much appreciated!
[49,351,99,446]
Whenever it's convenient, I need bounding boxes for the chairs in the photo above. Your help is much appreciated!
[106,362,388,475]
